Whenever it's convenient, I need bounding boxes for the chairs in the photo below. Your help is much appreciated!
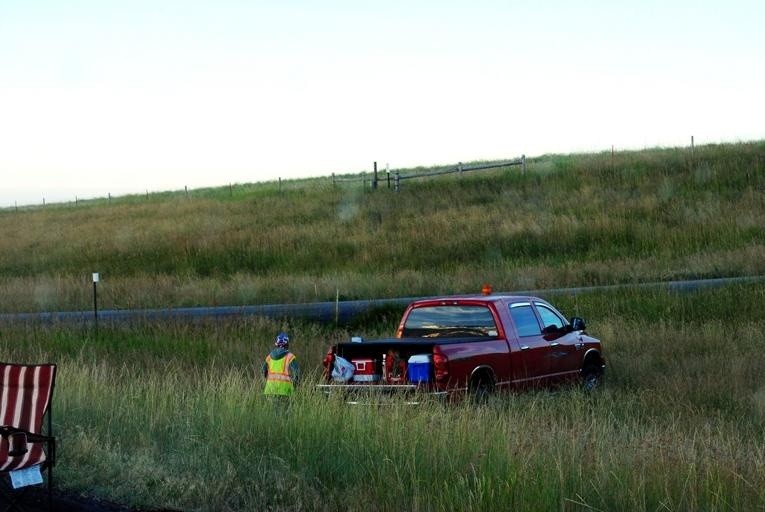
[0,362,57,496]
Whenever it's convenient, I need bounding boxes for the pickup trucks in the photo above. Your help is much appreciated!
[314,286,606,412]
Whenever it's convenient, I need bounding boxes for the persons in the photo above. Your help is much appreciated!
[261,331,299,414]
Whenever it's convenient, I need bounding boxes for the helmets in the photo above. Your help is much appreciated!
[275,333,289,347]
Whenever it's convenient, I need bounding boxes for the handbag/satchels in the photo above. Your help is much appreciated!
[332,354,355,383]
[386,350,407,385]
